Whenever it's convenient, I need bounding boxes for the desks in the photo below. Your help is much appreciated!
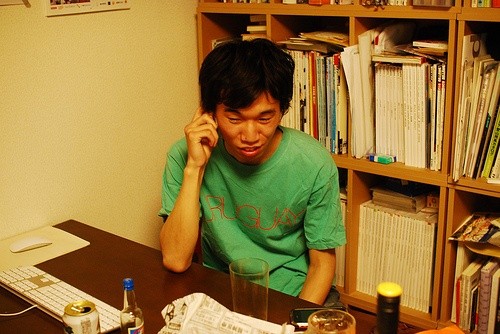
[0,220,414,334]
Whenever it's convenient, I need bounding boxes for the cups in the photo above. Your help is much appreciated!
[307,309,356,334]
[229,258,269,321]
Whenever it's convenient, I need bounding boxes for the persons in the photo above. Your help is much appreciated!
[158,38,345,313]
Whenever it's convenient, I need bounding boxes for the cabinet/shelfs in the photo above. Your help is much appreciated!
[196,0,500,334]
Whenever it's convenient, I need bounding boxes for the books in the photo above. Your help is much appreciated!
[213,0,500,334]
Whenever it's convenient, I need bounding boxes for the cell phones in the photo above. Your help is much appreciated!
[290,306,347,329]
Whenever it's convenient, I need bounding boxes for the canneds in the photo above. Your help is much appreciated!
[62,300,101,334]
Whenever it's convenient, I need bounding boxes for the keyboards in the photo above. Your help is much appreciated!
[0,264,134,334]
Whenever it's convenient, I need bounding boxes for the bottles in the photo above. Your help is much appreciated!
[377,283,402,334]
[120,278,144,334]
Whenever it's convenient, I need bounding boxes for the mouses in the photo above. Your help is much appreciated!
[9,237,53,253]
